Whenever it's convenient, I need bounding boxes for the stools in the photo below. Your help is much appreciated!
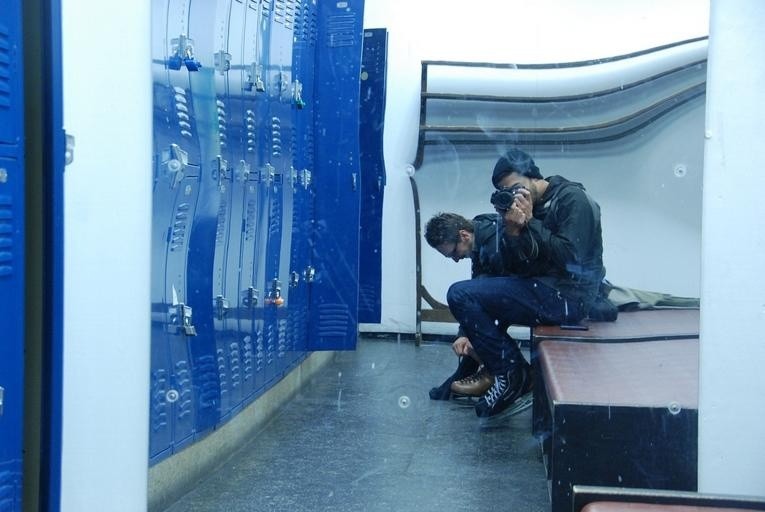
[530,338,700,512]
[530,308,700,365]
[571,485,765,512]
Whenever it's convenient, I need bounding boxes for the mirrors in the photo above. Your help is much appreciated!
[135,0,710,512]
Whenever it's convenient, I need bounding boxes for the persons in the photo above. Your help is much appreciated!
[447,150,607,417]
[425,212,521,400]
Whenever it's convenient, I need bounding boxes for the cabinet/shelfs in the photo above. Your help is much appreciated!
[149,1,389,468]
[0,0,67,512]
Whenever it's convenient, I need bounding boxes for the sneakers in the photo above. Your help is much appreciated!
[430,361,534,424]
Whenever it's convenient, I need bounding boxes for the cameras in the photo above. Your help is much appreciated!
[490,185,526,213]
